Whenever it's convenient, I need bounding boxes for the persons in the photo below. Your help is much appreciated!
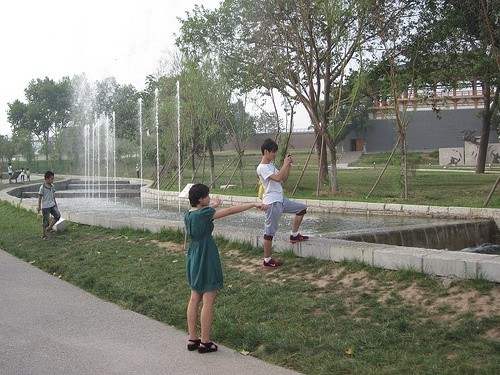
[36,171,61,241]
[183,184,269,354]
[20,167,31,182]
[136,163,140,178]
[256,139,307,267]
[8,163,14,183]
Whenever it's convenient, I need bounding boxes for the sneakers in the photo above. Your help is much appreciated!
[289,233,309,243]
[263,259,283,268]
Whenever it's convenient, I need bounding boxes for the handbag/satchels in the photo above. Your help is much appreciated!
[257,180,264,199]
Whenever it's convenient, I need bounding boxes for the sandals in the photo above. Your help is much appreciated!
[41,236,47,241]
[47,228,55,233]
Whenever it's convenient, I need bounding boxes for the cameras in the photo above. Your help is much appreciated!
[288,155,291,157]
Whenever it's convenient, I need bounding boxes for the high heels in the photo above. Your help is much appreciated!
[187,339,201,351]
[198,342,218,353]
